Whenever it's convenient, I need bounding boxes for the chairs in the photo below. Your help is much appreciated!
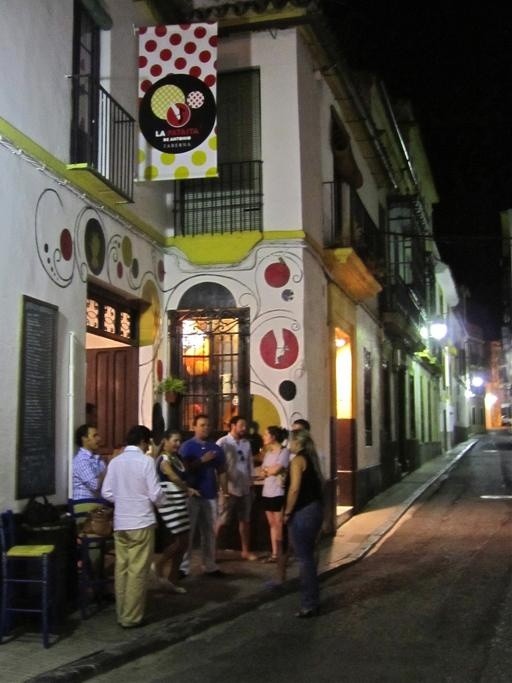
[0,497,115,652]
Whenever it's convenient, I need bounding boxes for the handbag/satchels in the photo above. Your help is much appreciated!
[25,494,60,524]
[154,511,174,554]
[81,506,113,537]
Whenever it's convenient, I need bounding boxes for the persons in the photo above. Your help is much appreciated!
[214,414,259,561]
[69,423,117,610]
[255,423,292,564]
[173,413,230,580]
[287,419,323,481]
[280,427,329,618]
[99,423,170,629]
[145,424,201,595]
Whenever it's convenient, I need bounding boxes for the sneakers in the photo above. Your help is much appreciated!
[239,552,283,564]
[151,568,229,595]
[294,608,318,617]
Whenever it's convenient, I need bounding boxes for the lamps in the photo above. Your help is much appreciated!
[429,313,448,341]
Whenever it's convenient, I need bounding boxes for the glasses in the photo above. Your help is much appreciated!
[238,450,245,461]
